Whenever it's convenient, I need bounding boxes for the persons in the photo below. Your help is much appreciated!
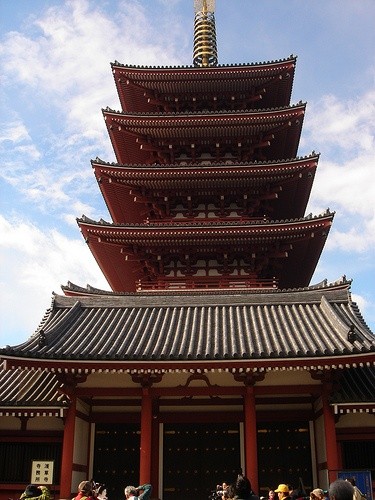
[260,489,277,500]
[125,484,151,500]
[345,477,369,500]
[232,475,259,500]
[209,483,235,500]
[20,485,51,500]
[89,478,110,500]
[329,480,354,500]
[72,481,97,500]
[309,489,329,500]
[275,484,294,500]
[289,490,299,500]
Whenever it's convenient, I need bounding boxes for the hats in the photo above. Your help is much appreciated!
[78,481,92,493]
[275,484,289,492]
[312,489,324,495]
